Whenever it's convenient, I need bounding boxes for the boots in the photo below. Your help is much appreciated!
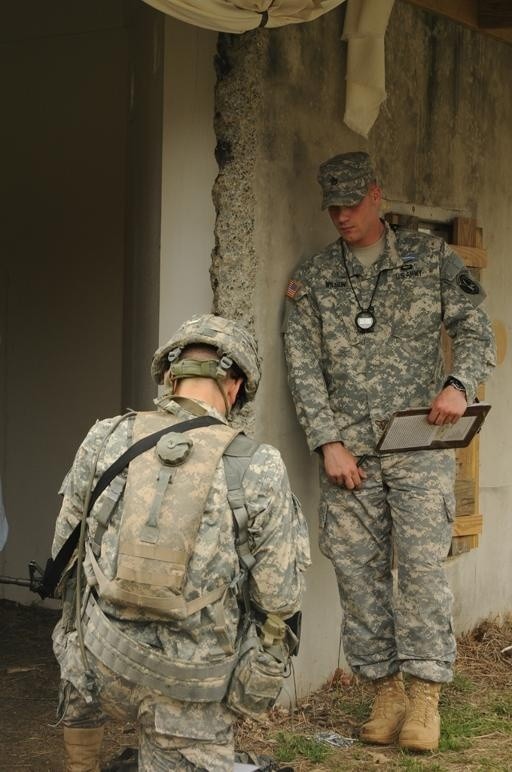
[400,675,442,748]
[359,673,408,743]
[63,726,104,771]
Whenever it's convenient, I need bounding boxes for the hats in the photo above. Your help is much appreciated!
[318,152,376,209]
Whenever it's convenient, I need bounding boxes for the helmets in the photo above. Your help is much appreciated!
[151,312,261,403]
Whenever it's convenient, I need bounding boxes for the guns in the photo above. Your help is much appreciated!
[1,561,303,659]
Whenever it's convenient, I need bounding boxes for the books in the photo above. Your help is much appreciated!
[377,401,491,456]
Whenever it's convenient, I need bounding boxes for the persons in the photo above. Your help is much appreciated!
[279,151,497,753]
[50,313,311,772]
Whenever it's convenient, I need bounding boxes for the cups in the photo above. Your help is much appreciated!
[261,618,286,650]
